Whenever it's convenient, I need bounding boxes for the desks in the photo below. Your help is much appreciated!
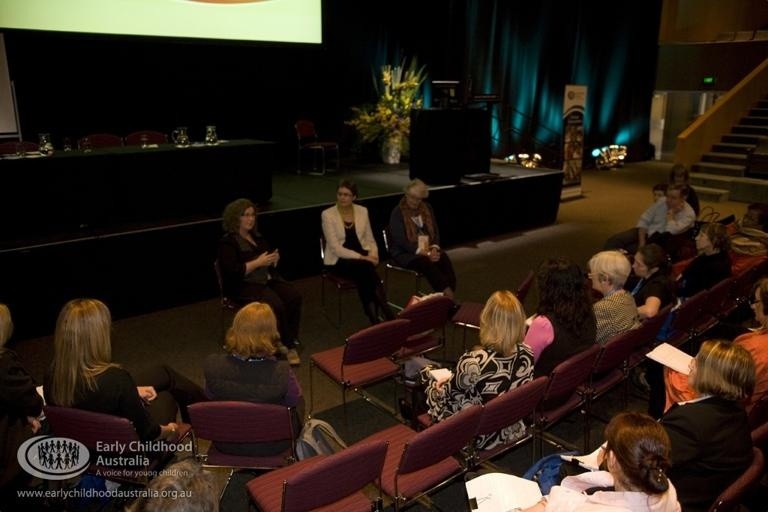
[0,137,276,246]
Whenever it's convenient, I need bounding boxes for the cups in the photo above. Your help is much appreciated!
[141,134,149,151]
[63,137,72,153]
[82,137,92,153]
[16,142,26,157]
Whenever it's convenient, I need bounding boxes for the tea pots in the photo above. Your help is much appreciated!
[38,132,55,158]
[205,125,218,146]
[172,127,191,148]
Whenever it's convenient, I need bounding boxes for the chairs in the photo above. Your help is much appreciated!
[77,134,124,153]
[0,140,40,156]
[124,130,168,146]
[296,118,340,177]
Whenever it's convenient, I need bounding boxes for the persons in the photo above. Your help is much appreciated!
[1,164,766,510]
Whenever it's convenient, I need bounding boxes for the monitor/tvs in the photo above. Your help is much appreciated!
[431,78,466,108]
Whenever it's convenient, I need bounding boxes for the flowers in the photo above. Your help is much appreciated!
[344,48,428,157]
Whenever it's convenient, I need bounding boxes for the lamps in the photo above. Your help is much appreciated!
[504,153,543,169]
[592,144,627,171]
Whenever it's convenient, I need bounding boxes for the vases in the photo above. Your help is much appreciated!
[381,130,402,165]
